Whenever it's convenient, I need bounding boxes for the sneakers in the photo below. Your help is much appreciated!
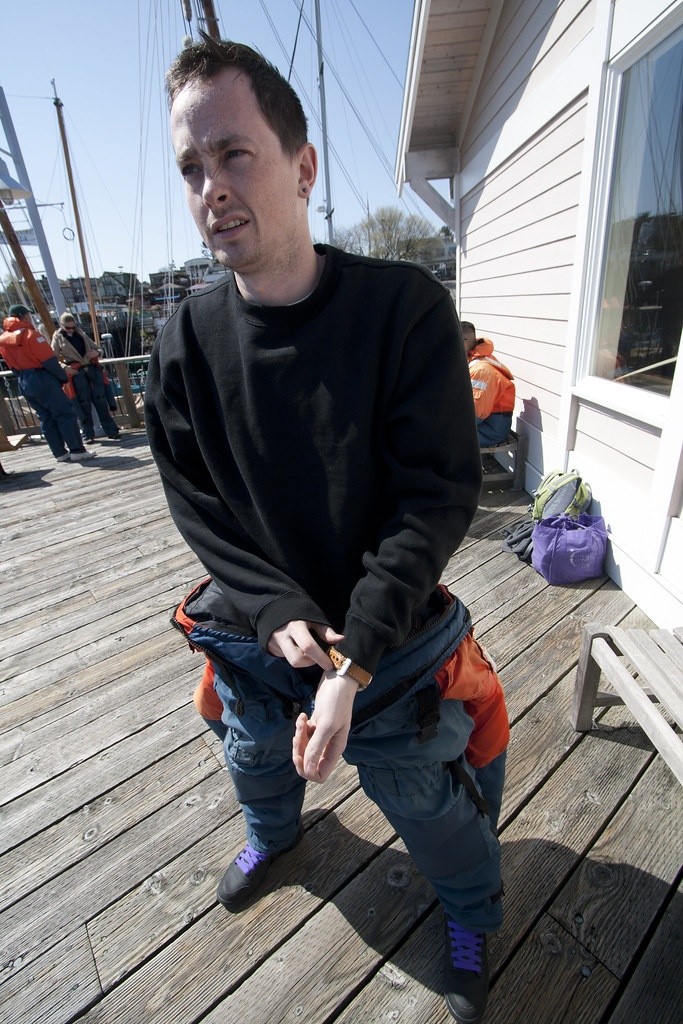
[217,821,304,908]
[438,892,489,1022]
[56,450,96,462]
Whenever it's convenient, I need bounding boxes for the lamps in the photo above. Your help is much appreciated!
[0,158,32,205]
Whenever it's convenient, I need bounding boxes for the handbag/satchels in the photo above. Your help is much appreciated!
[503,514,609,586]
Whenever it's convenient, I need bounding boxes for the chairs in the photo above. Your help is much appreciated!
[0,426,29,480]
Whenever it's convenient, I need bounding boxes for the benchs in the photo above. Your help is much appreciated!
[479,429,527,492]
[568,622,683,788]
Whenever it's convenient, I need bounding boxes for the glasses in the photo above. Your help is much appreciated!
[61,324,78,330]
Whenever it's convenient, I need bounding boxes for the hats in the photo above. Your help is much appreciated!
[9,304,34,315]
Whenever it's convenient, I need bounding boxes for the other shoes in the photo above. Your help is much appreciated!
[108,432,121,439]
[82,435,93,444]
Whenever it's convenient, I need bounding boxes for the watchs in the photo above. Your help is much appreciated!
[324,645,374,693]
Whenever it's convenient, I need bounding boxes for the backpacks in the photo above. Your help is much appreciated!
[528,469,590,526]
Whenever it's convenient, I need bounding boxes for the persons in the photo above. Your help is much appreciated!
[96,316,107,340]
[144,28,506,1024]
[0,304,97,461]
[50,312,123,445]
[459,320,514,448]
